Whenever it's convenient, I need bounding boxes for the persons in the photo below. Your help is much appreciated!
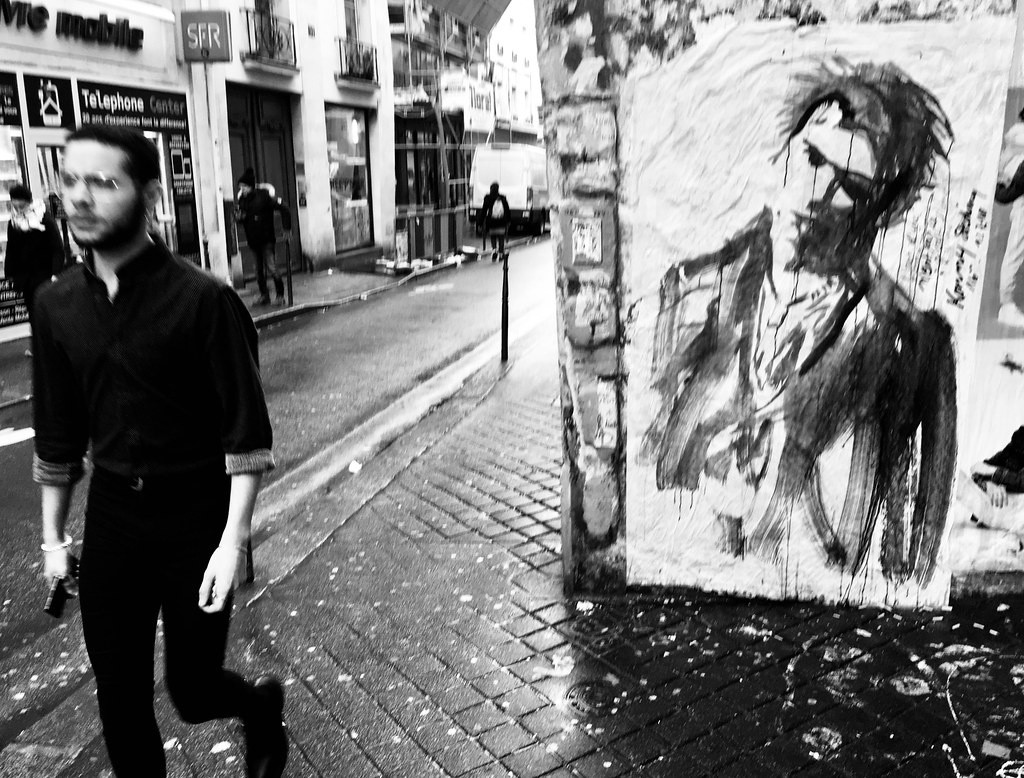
[3,185,66,355]
[481,182,510,262]
[30,123,290,778]
[970,107,1024,532]
[233,166,292,308]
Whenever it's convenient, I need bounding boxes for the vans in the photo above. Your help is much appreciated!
[468,141,553,236]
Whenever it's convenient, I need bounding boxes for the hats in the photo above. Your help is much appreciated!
[236,166,256,189]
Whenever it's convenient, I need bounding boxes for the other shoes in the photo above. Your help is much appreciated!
[241,674,291,778]
[498,248,509,262]
[23,348,35,358]
[491,251,498,263]
[251,294,271,309]
[271,296,285,309]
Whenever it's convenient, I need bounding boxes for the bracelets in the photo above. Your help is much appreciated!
[41,536,73,552]
[217,542,249,556]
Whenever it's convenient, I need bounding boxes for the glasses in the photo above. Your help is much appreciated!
[55,169,146,200]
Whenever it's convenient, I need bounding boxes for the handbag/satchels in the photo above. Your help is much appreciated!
[490,197,506,220]
